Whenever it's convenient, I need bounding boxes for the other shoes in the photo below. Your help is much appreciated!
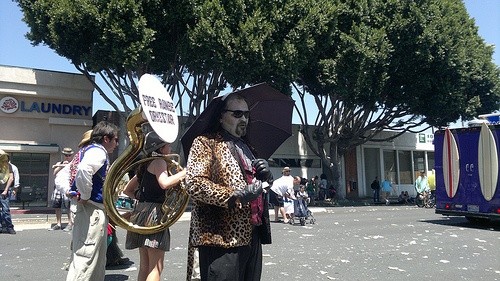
[48,224,61,231]
[111,258,129,267]
[386,200,388,205]
[7,227,16,234]
[63,228,70,232]
[284,218,290,224]
[275,219,281,223]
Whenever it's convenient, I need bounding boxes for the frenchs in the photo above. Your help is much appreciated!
[102,73,190,235]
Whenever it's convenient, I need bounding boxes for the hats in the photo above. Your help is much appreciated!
[142,130,166,155]
[61,148,74,155]
[79,130,93,147]
[282,167,292,174]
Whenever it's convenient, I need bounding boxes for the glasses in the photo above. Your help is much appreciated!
[64,154,72,157]
[109,136,118,142]
[221,110,250,119]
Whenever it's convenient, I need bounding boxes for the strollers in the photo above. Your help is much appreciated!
[288,190,316,226]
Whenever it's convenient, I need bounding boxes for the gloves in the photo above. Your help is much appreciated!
[252,159,271,181]
[236,180,262,204]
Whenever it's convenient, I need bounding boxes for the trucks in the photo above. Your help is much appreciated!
[434,112,500,225]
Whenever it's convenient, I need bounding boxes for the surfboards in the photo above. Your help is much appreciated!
[477,121,498,201]
[443,129,460,198]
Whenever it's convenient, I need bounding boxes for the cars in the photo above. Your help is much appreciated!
[19,186,33,201]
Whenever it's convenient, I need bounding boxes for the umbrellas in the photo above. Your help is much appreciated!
[179,81,296,158]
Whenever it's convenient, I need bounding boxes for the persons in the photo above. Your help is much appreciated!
[297,184,308,208]
[380,179,393,205]
[50,121,120,281]
[124,133,187,281]
[428,169,436,204]
[372,176,381,202]
[0,150,20,234]
[398,191,413,205]
[302,175,336,201]
[415,170,429,206]
[186,97,274,281]
[282,167,295,217]
[269,176,301,224]
